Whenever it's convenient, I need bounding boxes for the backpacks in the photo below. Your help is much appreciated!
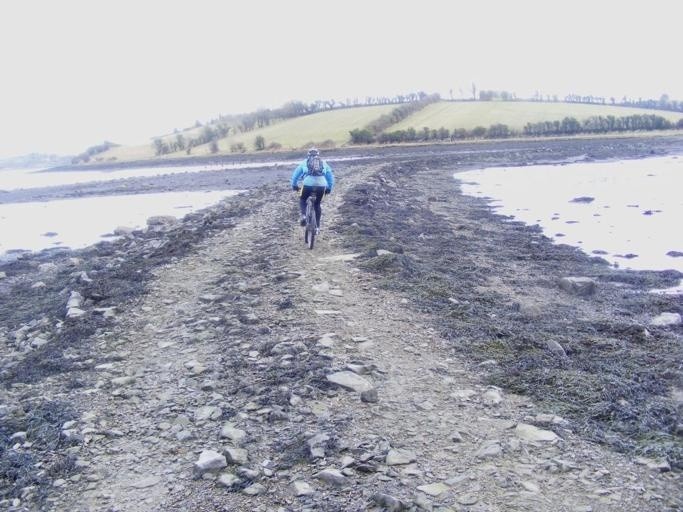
[307,156,324,176]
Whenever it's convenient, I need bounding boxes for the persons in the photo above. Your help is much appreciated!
[291,147,334,234]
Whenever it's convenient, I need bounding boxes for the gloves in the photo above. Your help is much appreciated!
[292,186,298,191]
[325,187,330,195]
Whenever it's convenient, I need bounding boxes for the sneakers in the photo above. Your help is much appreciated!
[301,216,307,227]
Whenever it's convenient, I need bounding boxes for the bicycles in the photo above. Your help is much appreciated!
[294,187,329,250]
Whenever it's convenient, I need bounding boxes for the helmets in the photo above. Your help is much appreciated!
[307,149,320,155]
